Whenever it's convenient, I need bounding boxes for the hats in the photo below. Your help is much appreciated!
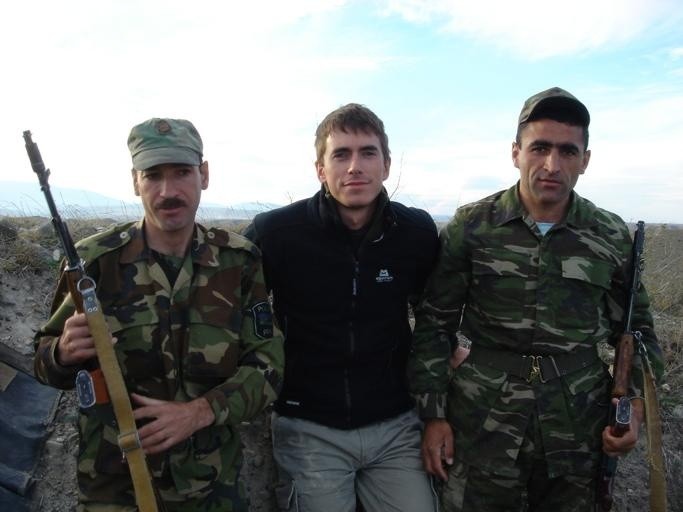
[519,87,590,126]
[127,117,207,171]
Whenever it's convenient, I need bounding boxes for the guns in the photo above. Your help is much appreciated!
[601,221,644,512]
[22,129,166,512]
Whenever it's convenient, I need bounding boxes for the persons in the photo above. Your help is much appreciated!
[242,103,470,512]
[33,118,286,511]
[406,87,664,512]
